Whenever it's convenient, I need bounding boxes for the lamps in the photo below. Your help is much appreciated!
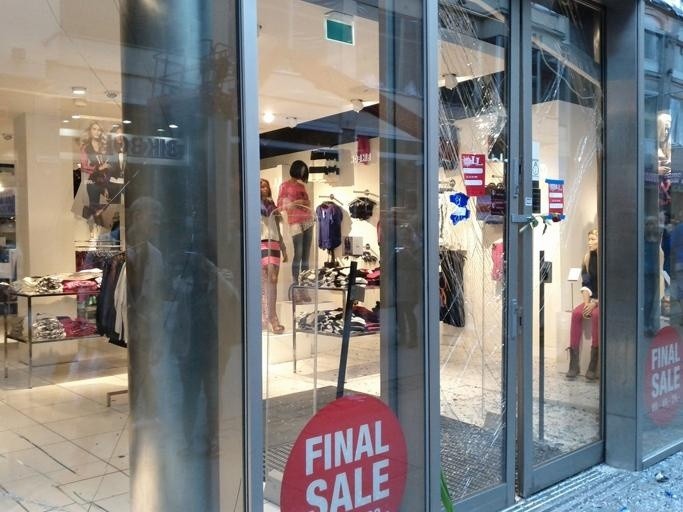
[444,73,458,89]
[350,98,364,114]
[285,116,297,129]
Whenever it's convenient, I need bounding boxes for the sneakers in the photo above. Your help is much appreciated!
[660,294,671,317]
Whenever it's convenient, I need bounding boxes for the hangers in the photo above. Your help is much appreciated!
[74,245,128,266]
[316,194,344,211]
[347,189,380,207]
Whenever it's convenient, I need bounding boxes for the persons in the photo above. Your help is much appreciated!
[80,121,111,220]
[60,195,179,400]
[658,112,673,205]
[645,209,682,336]
[260,178,287,334]
[140,216,239,456]
[564,227,600,381]
[277,159,316,302]
[99,125,125,204]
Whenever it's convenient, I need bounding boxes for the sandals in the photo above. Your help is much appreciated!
[301,288,312,302]
[294,288,302,305]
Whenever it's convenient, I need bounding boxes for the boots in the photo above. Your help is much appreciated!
[585,345,599,380]
[564,346,581,377]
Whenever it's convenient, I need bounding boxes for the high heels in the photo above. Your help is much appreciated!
[265,313,286,334]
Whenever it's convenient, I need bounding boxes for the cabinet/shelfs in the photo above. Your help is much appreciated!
[284,273,380,378]
[0,281,112,391]
